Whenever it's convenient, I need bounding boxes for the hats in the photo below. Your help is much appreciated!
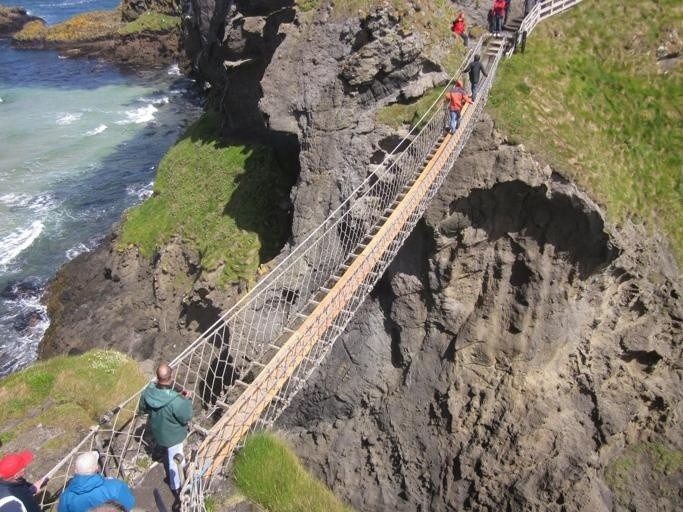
[0,451,33,480]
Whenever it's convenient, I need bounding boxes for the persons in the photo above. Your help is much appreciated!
[462,54,488,101]
[139,363,193,498]
[451,11,469,47]
[487,0,511,37]
[0,450,137,512]
[445,80,474,134]
